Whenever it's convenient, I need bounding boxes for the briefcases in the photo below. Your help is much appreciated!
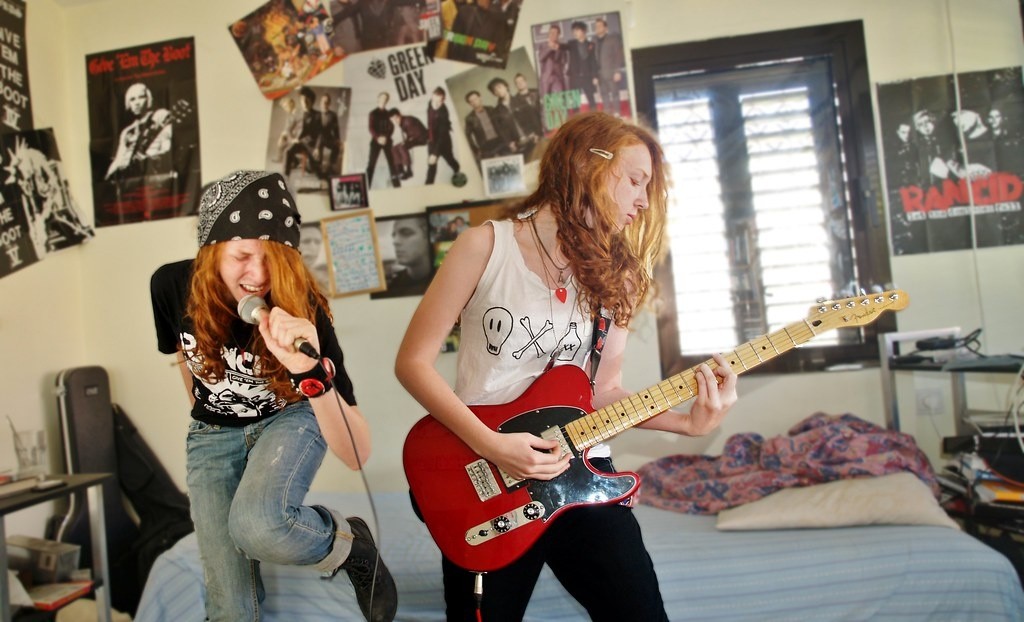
[49,363,138,611]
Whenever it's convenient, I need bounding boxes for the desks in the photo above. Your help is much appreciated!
[877,325,1024,433]
[0,473,117,622]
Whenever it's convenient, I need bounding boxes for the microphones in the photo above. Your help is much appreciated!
[238,293,320,361]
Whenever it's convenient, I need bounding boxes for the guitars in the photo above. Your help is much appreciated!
[403,287,911,573]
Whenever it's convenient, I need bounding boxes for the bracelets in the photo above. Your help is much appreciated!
[284,358,337,400]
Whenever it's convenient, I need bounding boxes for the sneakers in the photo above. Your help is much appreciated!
[337,515,399,622]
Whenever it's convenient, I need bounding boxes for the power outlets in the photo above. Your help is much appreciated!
[914,388,944,416]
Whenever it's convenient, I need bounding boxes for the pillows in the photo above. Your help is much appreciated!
[715,470,960,530]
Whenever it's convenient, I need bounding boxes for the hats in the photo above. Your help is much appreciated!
[196,169,302,249]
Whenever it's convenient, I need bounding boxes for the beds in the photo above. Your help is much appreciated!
[134,487,1024,622]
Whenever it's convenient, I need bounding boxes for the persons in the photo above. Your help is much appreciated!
[106,84,194,217]
[537,19,622,121]
[395,110,738,622]
[895,102,1024,250]
[274,74,557,185]
[150,169,401,621]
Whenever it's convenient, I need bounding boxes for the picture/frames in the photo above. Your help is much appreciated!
[328,173,369,212]
[480,153,527,196]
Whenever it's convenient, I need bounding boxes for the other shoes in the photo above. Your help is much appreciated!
[393,165,403,178]
[399,165,413,178]
[425,179,433,184]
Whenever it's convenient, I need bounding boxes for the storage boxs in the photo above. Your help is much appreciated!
[7,533,80,579]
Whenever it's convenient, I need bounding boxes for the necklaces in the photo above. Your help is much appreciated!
[527,207,582,351]
